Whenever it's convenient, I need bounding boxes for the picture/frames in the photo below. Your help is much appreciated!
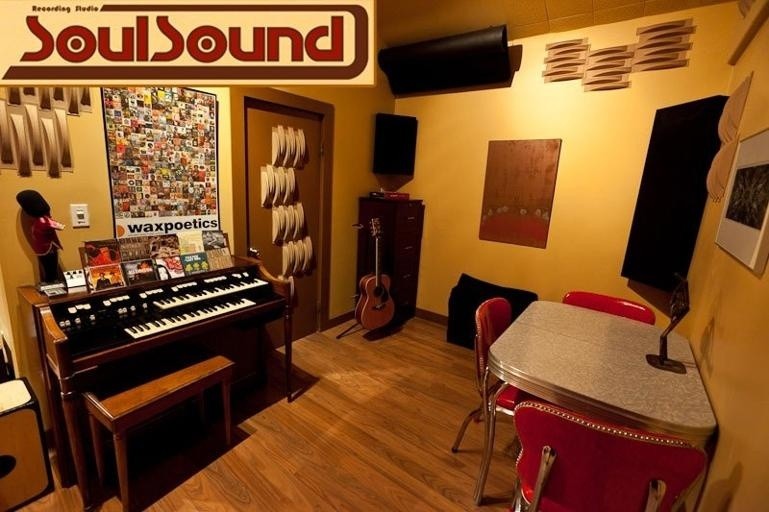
[715,127,769,278]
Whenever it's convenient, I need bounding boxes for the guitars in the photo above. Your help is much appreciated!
[354,218,396,330]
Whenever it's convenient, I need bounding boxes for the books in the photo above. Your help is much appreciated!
[78,230,231,294]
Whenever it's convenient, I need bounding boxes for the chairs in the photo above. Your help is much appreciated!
[562,291,656,326]
[510,400,708,512]
[452,297,551,453]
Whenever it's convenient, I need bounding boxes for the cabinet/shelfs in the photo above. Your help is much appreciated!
[356,196,423,324]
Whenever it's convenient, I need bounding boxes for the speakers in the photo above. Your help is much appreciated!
[372,112,417,176]
[377,24,511,95]
[621,95,731,293]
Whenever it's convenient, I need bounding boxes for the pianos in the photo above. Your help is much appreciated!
[17,253,291,508]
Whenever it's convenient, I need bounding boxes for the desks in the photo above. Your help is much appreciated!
[473,300,717,507]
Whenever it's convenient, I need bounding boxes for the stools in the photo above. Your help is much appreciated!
[81,347,236,512]
[0,376,54,512]
